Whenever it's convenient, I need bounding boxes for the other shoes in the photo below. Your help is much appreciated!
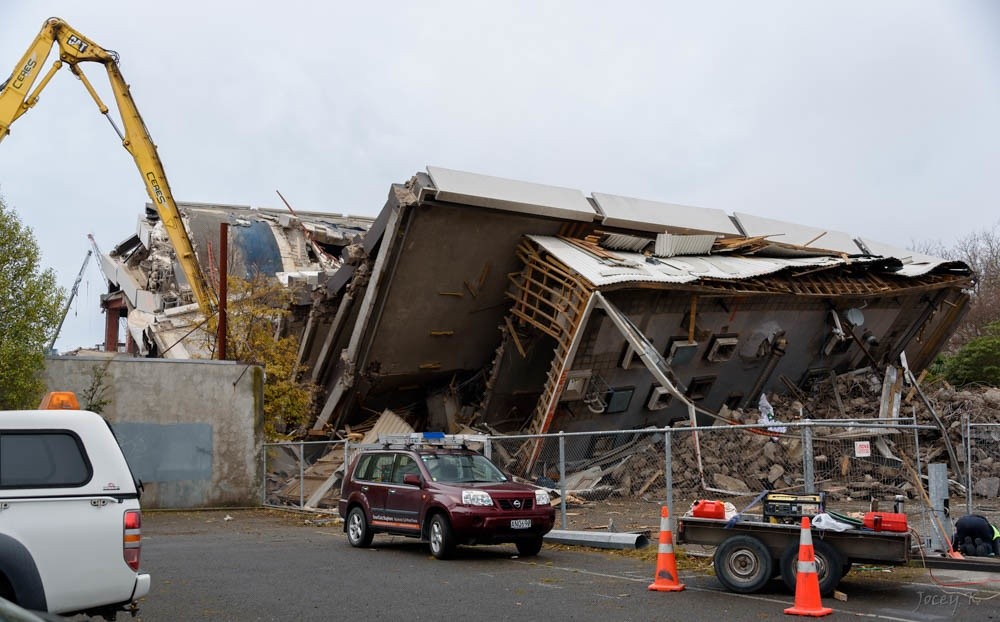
[975,538,986,556]
[965,536,975,556]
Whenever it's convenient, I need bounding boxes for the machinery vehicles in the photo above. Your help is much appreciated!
[0,14,231,359]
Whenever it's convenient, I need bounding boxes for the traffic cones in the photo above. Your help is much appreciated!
[783,517,833,618]
[646,505,687,592]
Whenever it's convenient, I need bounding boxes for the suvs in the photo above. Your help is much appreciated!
[1,389,151,622]
[337,431,557,562]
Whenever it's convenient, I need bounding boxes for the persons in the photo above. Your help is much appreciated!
[951,514,1000,557]
